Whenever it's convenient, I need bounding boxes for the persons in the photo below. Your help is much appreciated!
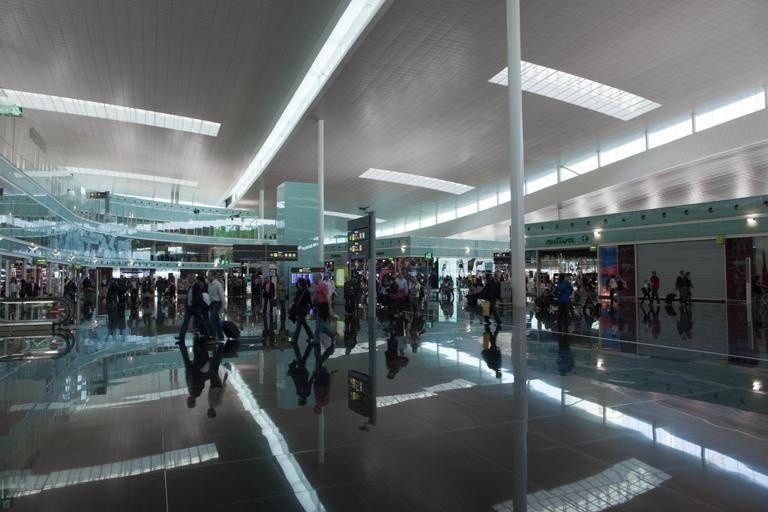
[206,342,229,420]
[344,272,426,318]
[478,269,508,325]
[384,329,409,381]
[407,310,426,354]
[342,316,361,357]
[228,270,339,346]
[649,305,661,339]
[526,272,600,331]
[438,274,484,304]
[649,270,660,304]
[673,306,688,342]
[607,274,627,308]
[672,270,686,304]
[174,335,212,408]
[286,338,314,408]
[278,315,291,352]
[555,332,575,378]
[679,270,695,306]
[685,306,694,339]
[261,312,277,353]
[310,339,339,414]
[2,275,176,334]
[480,321,502,378]
[175,269,226,346]
[639,304,653,335]
[640,278,651,304]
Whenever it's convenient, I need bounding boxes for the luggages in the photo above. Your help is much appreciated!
[665,291,676,305]
[219,339,241,359]
[219,310,241,339]
[665,304,678,317]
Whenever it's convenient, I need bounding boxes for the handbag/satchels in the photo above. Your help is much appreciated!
[482,331,491,352]
[481,301,491,317]
[270,299,278,308]
